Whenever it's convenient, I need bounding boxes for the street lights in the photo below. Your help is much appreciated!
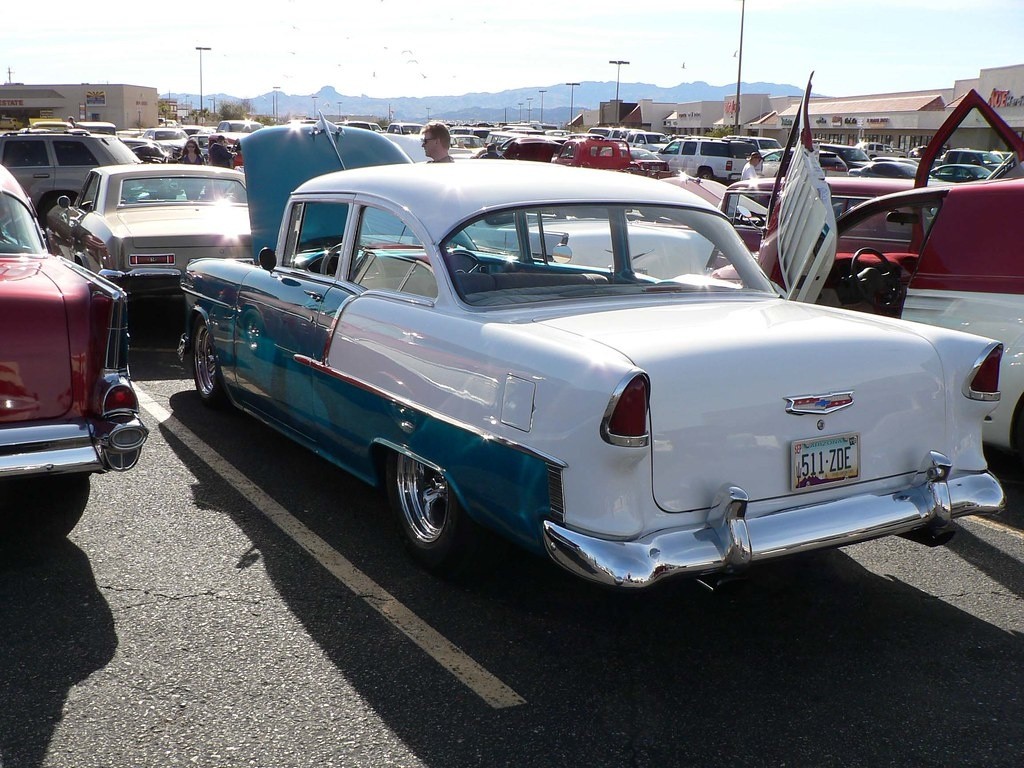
[312,96,319,119]
[336,101,343,122]
[565,82,581,132]
[608,60,631,127]
[272,87,282,121]
[526,97,533,123]
[518,103,523,121]
[194,46,211,126]
[538,90,548,123]
[426,106,431,124]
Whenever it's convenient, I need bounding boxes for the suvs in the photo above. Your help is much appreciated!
[0,129,147,233]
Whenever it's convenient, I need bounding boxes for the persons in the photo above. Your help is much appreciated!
[210,135,237,168]
[68,116,76,128]
[911,148,922,158]
[457,140,464,147]
[740,152,763,180]
[470,143,501,159]
[232,143,243,169]
[179,140,206,200]
[416,122,454,163]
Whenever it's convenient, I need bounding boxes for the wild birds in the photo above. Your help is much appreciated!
[383,47,428,79]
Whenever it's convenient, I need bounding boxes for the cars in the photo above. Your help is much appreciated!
[0,163,149,481]
[40,161,255,302]
[709,175,1024,474]
[0,117,24,131]
[449,117,1024,273]
[177,111,1010,592]
[20,117,424,169]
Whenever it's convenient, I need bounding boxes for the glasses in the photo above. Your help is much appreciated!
[187,146,195,148]
[757,158,761,162]
[422,139,436,145]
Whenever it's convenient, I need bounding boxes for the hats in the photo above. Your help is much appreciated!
[216,136,227,140]
[746,152,765,161]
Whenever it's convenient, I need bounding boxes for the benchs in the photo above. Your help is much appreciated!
[454,271,609,295]
